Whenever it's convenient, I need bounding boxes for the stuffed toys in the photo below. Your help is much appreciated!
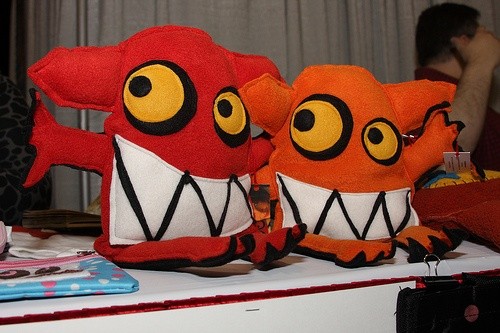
[24,24,307,269]
[234,64,470,268]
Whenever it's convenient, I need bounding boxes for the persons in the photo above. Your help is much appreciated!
[412,2,498,175]
